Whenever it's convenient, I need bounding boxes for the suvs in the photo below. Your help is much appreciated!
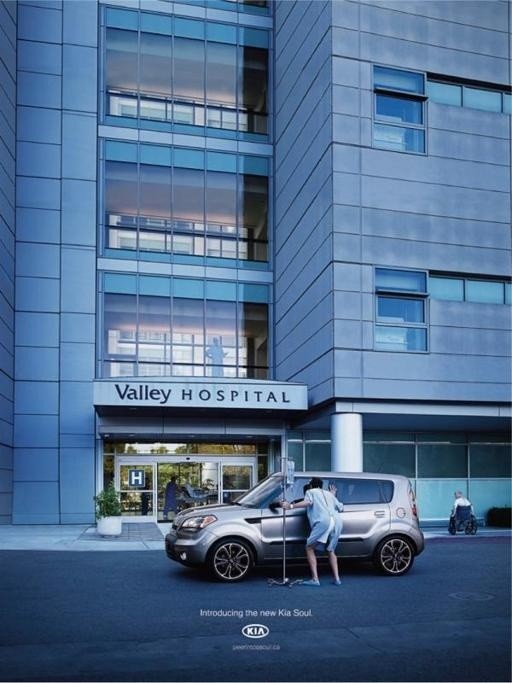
[164,470,426,584]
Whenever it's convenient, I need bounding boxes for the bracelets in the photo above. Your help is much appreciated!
[289,503,293,509]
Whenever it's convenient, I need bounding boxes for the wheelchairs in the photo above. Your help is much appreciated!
[447,504,478,536]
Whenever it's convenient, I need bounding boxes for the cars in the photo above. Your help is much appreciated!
[155,484,226,510]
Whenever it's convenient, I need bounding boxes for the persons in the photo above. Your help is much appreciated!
[448,489,475,532]
[140,476,150,515]
[163,474,179,519]
[205,335,230,376]
[279,475,345,586]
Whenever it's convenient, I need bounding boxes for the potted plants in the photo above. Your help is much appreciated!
[93,481,122,536]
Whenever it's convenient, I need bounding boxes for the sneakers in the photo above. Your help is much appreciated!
[334,581,342,586]
[303,580,319,586]
